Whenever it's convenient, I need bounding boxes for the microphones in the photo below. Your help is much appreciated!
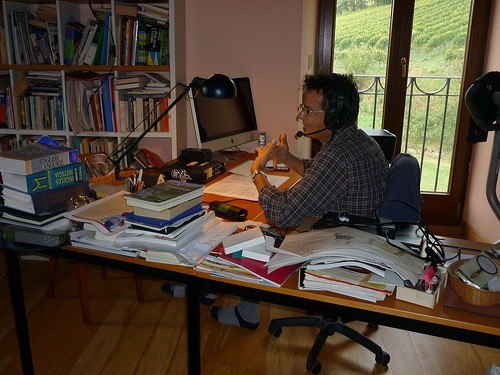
[297,120,339,137]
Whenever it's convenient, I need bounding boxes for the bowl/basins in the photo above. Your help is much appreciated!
[447,258,500,307]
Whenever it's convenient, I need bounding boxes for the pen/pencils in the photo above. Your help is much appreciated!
[274,167,290,171]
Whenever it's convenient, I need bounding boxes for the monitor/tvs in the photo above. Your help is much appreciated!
[188,76,260,152]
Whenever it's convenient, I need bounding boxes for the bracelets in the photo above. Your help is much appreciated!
[251,169,267,183]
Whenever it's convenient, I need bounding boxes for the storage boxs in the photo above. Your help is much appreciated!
[222,226,265,255]
[242,235,275,262]
[396,266,448,309]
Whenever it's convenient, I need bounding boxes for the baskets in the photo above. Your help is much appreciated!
[448,259,500,307]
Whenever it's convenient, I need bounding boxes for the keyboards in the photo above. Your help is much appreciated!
[229,159,254,176]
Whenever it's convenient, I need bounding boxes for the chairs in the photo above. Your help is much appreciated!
[269,153,422,375]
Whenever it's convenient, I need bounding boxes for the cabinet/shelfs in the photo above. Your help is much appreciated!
[0,0,187,170]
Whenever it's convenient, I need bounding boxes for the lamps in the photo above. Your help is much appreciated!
[106,74,237,179]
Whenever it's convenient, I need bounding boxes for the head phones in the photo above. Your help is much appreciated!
[324,77,346,130]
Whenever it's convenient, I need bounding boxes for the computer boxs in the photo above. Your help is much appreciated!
[297,128,396,163]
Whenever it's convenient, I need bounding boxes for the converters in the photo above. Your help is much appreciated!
[378,216,396,237]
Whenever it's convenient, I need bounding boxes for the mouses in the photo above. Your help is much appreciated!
[338,209,350,223]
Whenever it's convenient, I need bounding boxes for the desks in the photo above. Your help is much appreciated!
[0,151,500,375]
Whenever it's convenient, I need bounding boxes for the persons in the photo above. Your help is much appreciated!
[160,70,390,329]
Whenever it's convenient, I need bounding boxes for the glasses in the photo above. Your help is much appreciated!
[300,104,326,117]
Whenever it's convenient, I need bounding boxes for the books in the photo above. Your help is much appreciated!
[0,133,161,227]
[204,173,290,202]
[0,3,170,65]
[0,71,171,132]
[64,179,429,304]
[265,160,290,172]
